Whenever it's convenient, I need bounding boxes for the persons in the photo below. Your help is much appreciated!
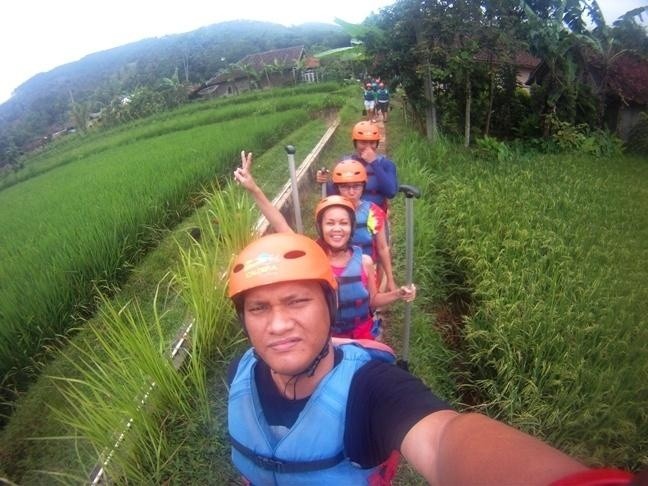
[232,149,416,342]
[332,158,399,294]
[360,72,390,123]
[224,232,647,486]
[317,121,398,265]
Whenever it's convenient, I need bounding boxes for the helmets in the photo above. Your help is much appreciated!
[332,159,368,192]
[366,79,385,88]
[352,121,381,152]
[226,231,337,322]
[314,194,356,239]
